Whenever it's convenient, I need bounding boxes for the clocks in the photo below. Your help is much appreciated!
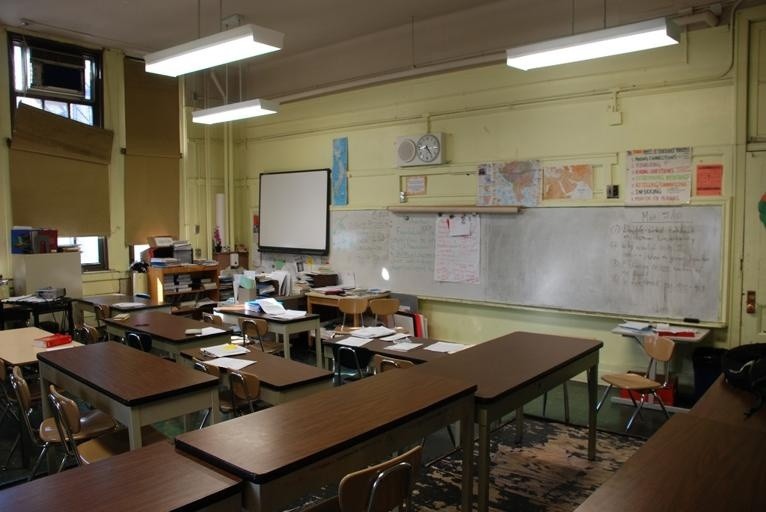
[415,133,440,163]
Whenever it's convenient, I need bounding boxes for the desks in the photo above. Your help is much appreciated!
[413,331,605,512]
[611,321,709,413]
[1,264,480,511]
[571,370,765,512]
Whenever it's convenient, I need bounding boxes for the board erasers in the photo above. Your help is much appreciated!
[683,318,700,323]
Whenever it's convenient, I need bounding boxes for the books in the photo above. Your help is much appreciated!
[618,320,696,337]
[32,333,74,348]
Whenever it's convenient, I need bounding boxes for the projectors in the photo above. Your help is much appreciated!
[36,287,67,299]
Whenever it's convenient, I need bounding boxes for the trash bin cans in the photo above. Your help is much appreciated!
[692,347,729,403]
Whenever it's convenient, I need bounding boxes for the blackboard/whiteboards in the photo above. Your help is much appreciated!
[327,197,733,328]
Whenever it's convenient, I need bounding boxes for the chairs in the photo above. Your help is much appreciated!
[596,334,675,433]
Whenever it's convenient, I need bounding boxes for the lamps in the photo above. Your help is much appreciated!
[141,0,285,79]
[504,0,681,73]
[190,58,279,125]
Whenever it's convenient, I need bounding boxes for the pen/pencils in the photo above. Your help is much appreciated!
[135,324,149,326]
